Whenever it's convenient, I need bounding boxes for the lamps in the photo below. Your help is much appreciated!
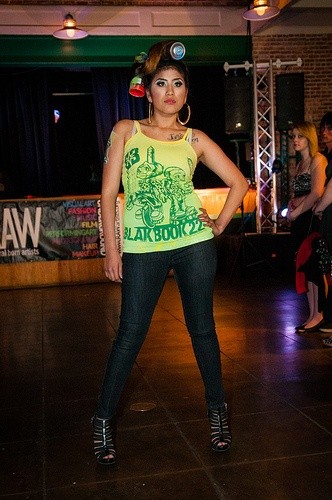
[53,11,89,40]
[242,0,280,21]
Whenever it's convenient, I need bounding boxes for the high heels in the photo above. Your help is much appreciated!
[90,414,116,465]
[207,400,232,451]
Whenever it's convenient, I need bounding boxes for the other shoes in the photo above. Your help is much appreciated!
[320,336,332,347]
[295,319,325,333]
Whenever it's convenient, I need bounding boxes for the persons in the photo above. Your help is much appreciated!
[89,39,249,464]
[288,111,332,347]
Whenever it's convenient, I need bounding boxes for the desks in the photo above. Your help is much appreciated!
[195,187,256,230]
